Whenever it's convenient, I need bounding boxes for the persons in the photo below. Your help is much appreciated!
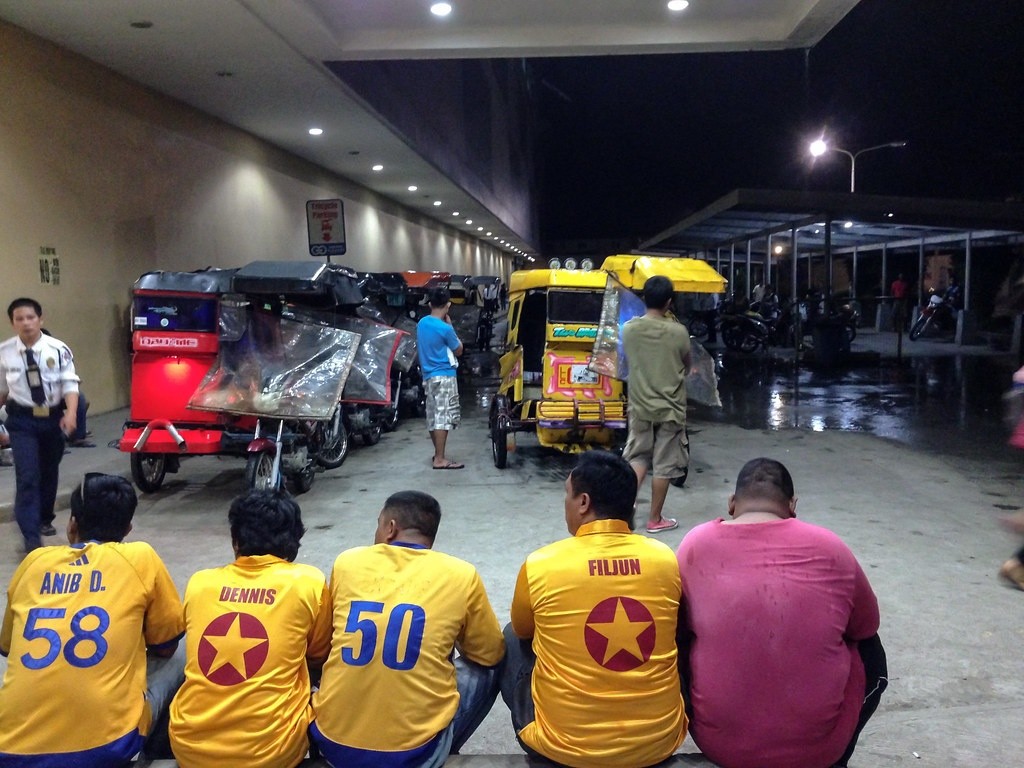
[499,284,508,310]
[621,276,694,534]
[675,457,890,768]
[59,393,97,447]
[308,490,506,768]
[945,277,960,299]
[0,298,82,556]
[0,474,186,768]
[416,291,465,469]
[167,490,333,768]
[751,276,764,302]
[890,271,907,331]
[483,285,494,316]
[762,284,774,303]
[502,449,689,768]
[704,292,718,344]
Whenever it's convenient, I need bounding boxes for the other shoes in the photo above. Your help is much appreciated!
[40,523,56,536]
[70,440,96,447]
[24,539,42,557]
[86,430,94,437]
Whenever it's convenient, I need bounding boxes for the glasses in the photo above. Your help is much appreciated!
[79,471,132,505]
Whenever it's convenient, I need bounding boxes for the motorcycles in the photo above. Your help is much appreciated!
[686,289,956,352]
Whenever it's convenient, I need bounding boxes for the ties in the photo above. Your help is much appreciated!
[25,349,46,408]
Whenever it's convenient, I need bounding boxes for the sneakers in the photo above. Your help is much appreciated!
[647,516,679,533]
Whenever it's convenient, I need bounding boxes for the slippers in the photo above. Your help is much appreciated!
[432,460,465,469]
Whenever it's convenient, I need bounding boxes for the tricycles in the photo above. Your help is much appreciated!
[115,255,728,500]
